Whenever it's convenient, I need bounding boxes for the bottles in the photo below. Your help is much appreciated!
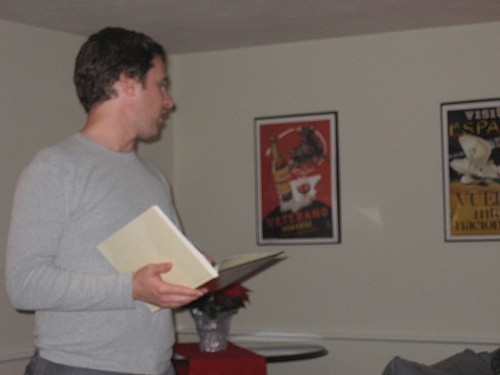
[269,136,292,212]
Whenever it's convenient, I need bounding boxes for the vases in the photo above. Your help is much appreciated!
[191,309,237,353]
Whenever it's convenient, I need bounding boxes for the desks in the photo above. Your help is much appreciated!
[172,341,323,375]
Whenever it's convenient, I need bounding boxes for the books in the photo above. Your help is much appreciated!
[96,205,286,314]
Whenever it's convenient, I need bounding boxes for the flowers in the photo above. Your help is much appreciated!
[201,278,251,319]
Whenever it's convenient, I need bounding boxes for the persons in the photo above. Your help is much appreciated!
[5,26,215,375]
